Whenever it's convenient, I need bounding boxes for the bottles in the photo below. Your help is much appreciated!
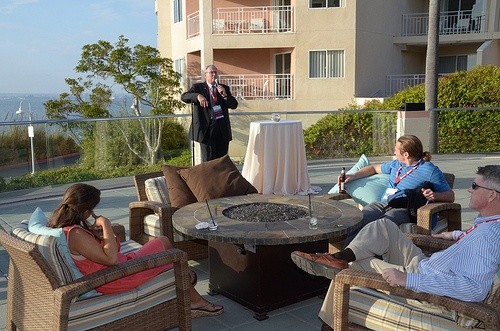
[339,168,347,193]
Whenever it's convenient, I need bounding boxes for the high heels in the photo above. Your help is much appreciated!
[191,303,225,319]
[188,270,197,286]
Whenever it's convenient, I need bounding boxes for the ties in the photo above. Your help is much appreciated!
[211,86,217,105]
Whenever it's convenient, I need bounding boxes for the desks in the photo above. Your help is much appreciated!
[396,111,430,154]
[227,20,246,33]
[241,120,322,195]
[229,85,248,101]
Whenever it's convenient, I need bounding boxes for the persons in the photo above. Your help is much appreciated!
[338,135,455,247]
[47,183,223,318]
[180,65,238,166]
[290,165,500,331]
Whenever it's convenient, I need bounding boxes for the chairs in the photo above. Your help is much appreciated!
[128,171,209,260]
[212,19,226,34]
[253,80,269,100]
[0,217,191,331]
[321,173,462,237]
[334,233,500,331]
[248,18,264,33]
[452,18,470,34]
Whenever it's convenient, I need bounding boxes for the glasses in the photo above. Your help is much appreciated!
[206,70,217,74]
[471,182,500,194]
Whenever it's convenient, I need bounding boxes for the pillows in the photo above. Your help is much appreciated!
[28,207,103,301]
[327,153,390,208]
[176,154,258,202]
[161,165,197,209]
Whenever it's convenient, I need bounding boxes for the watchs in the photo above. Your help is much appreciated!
[223,94,227,99]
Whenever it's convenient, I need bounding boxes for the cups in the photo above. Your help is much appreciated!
[207,205,218,231]
[308,204,319,230]
[271,113,281,122]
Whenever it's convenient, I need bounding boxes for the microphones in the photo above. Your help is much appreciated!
[215,79,223,94]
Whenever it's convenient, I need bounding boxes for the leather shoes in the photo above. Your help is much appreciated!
[291,251,348,280]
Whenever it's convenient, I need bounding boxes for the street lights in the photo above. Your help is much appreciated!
[16,99,35,175]
